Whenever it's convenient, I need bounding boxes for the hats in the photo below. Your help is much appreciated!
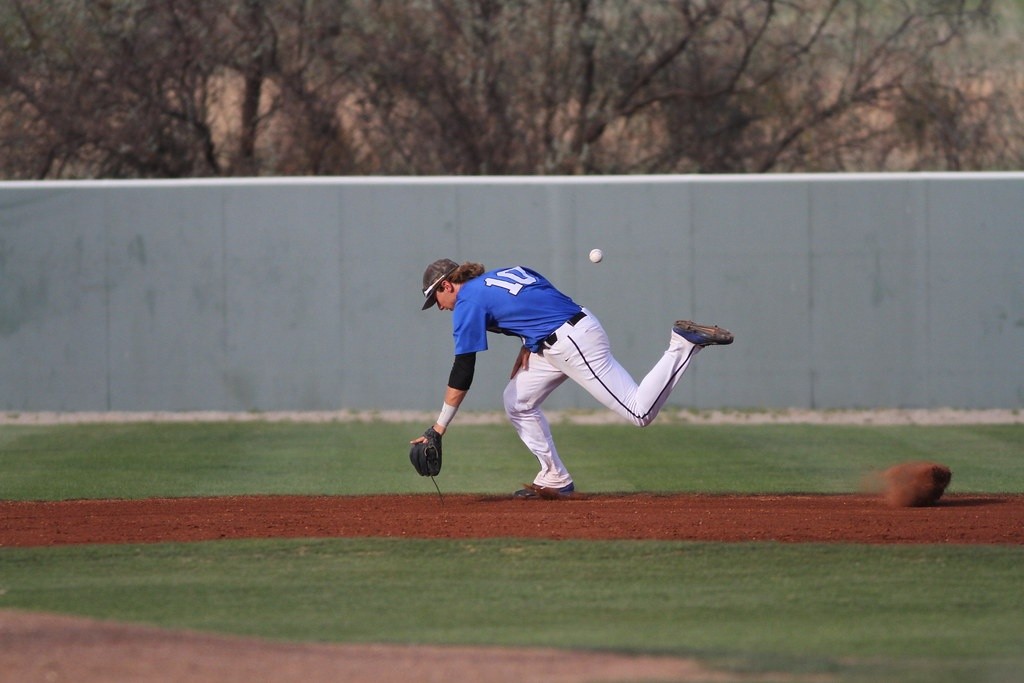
[422,257,459,310]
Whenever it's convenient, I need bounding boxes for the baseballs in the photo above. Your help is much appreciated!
[589,248,603,263]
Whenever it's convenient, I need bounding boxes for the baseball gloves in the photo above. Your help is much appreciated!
[409,426,443,477]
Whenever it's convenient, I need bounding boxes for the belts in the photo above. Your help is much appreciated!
[537,309,587,353]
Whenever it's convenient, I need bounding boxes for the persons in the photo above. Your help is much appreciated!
[410,258,734,500]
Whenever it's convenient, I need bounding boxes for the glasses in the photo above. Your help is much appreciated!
[423,275,444,298]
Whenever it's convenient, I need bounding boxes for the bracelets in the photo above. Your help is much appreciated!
[437,401,457,428]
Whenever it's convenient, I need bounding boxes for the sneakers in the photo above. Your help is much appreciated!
[514,482,575,498]
[672,320,734,348]
